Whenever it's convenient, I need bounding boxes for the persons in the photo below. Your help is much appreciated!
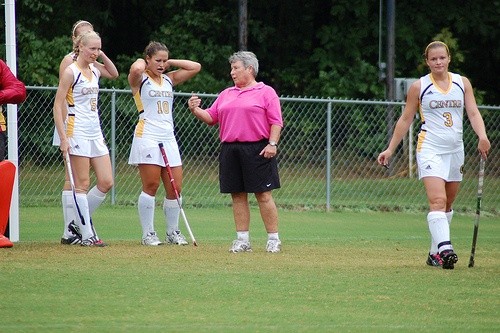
[378,41,491,269]
[0,60,26,162]
[53,20,118,247]
[188,51,283,254]
[128,41,201,246]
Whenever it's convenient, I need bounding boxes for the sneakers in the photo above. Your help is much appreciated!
[142,232,161,246]
[229,240,252,254]
[61,235,78,244]
[68,221,82,240]
[266,239,281,253]
[165,230,188,246]
[440,251,458,269]
[425,252,444,268]
[80,238,105,246]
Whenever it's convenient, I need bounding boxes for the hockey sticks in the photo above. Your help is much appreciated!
[159,142,198,248]
[468,154,486,267]
[64,148,87,226]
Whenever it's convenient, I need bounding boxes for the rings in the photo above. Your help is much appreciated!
[191,104,194,106]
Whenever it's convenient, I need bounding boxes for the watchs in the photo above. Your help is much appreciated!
[268,142,279,148]
